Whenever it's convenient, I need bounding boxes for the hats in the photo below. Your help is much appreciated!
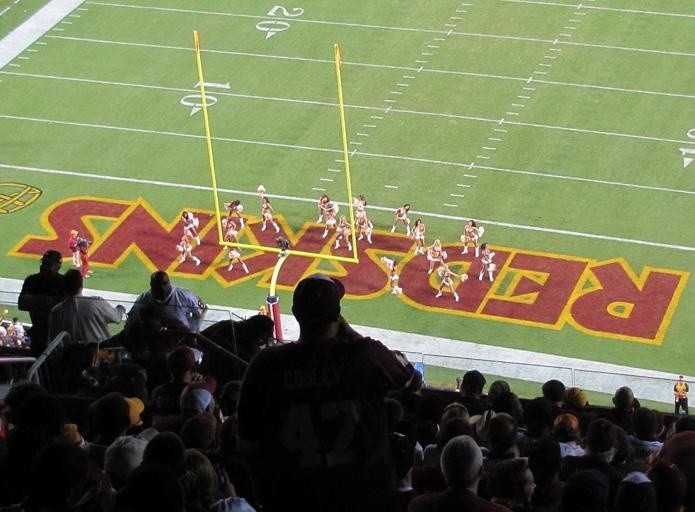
[292,272,344,306]
[76,346,218,485]
[562,388,587,408]
[554,413,579,432]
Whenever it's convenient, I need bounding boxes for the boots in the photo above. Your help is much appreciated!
[178,218,280,273]
[317,214,373,251]
[390,224,494,302]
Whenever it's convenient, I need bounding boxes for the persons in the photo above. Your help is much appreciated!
[315,194,499,247]
[0,247,693,510]
[69,230,80,268]
[180,183,280,273]
[77,237,94,277]
